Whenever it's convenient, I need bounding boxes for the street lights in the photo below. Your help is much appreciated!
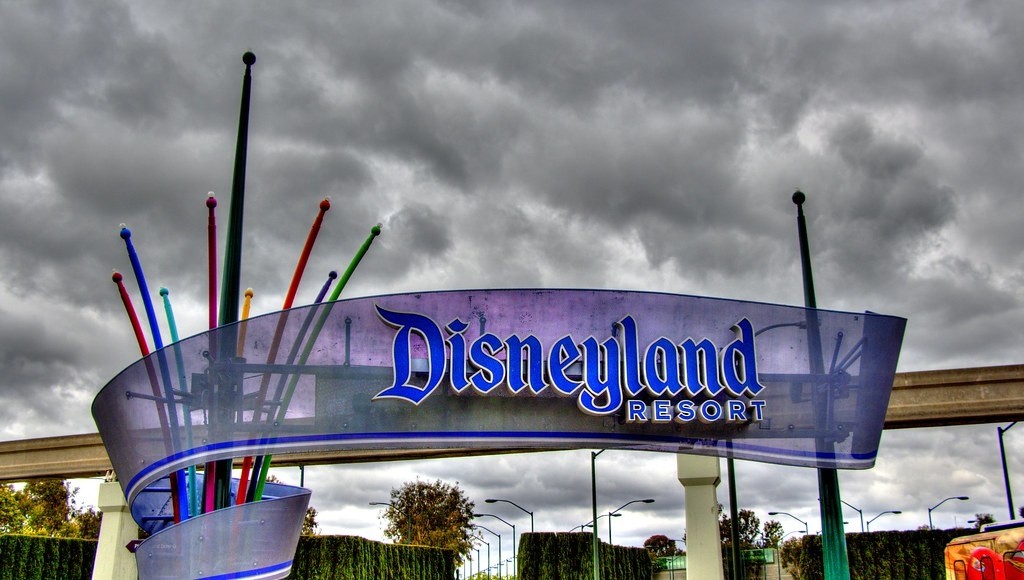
[608,498,655,545]
[368,502,410,545]
[485,499,533,535]
[569,523,594,533]
[818,497,864,533]
[726,319,849,580]
[966,520,988,526]
[866,510,902,531]
[460,513,516,580]
[581,513,622,533]
[928,496,969,531]
